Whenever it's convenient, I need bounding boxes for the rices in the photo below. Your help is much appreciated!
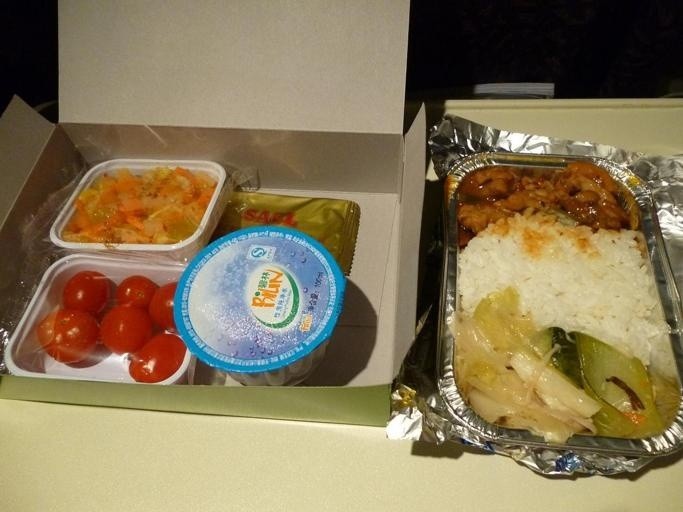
[457,206,659,369]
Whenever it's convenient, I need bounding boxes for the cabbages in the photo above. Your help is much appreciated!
[452,286,604,444]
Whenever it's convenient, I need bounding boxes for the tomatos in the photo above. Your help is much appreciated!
[37,269,186,382]
